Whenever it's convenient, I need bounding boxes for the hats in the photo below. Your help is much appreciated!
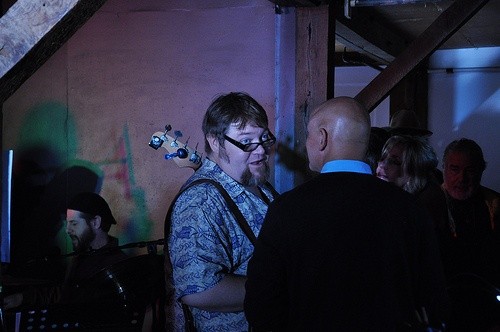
[381,109,434,136]
[67,193,117,224]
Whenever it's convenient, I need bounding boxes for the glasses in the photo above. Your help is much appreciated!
[224,128,276,152]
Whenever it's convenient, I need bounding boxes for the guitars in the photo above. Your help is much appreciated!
[148,124,203,172]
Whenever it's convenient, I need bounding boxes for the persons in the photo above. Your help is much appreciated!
[163,91,280,332]
[242,97,435,332]
[377,135,454,332]
[443,138,500,332]
[2,192,148,332]
